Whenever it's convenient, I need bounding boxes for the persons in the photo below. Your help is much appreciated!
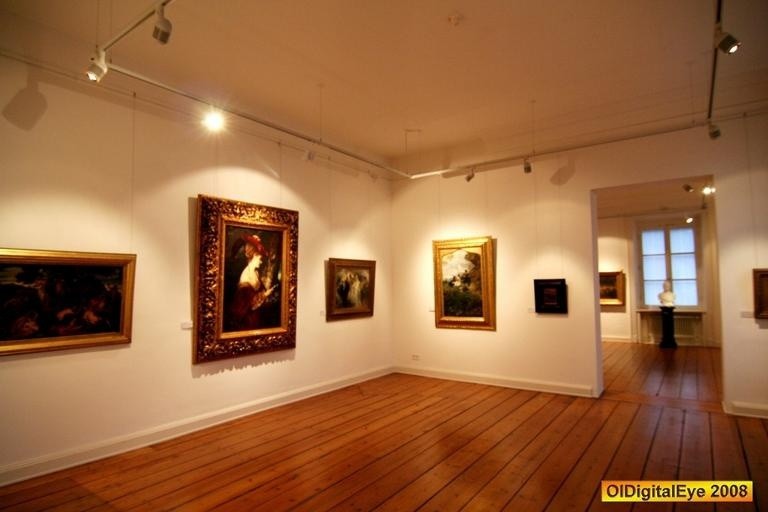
[0,265,122,342]
[658,281,675,305]
[335,270,370,307]
[449,252,481,296]
[230,233,277,330]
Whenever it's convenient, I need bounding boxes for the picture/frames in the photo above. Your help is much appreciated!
[431,235,497,332]
[533,278,568,314]
[192,193,299,367]
[0,247,137,359]
[326,257,376,322]
[598,271,626,308]
[753,268,768,319]
